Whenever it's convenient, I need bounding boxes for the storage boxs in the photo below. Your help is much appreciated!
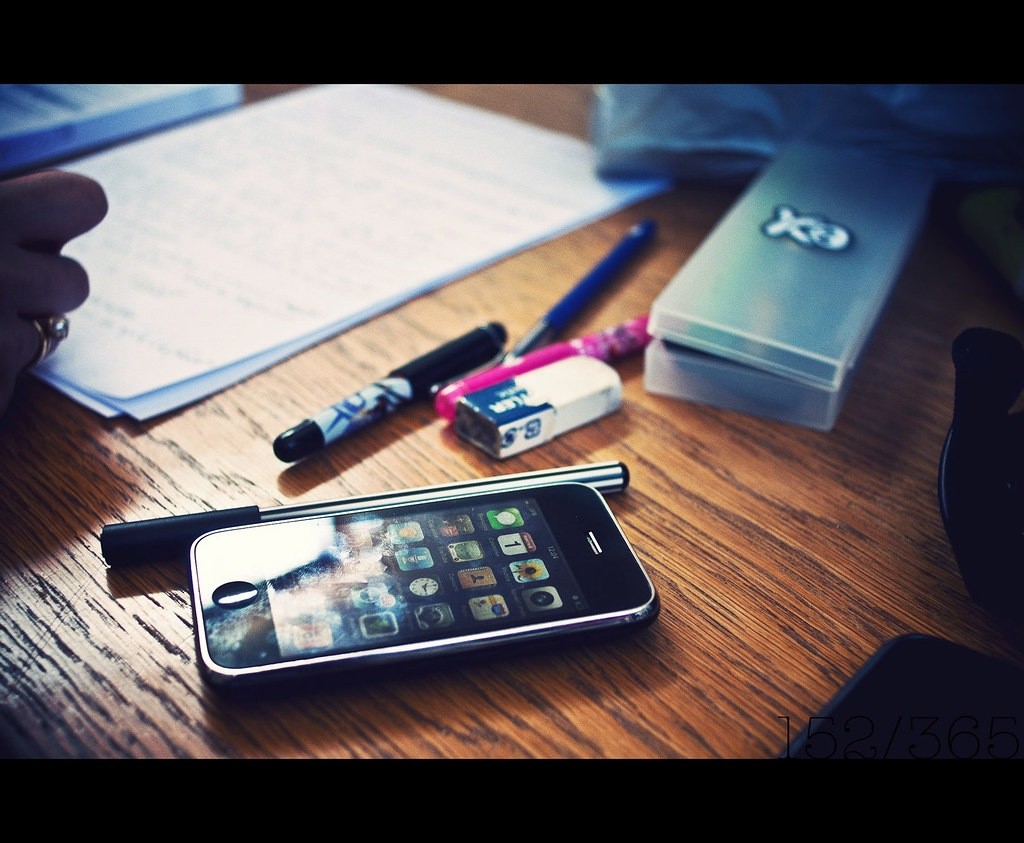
[645,141,938,431]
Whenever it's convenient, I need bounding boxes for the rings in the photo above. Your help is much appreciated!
[33,310,70,365]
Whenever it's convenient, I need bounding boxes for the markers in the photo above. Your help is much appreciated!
[432,313,659,423]
[274,320,508,464]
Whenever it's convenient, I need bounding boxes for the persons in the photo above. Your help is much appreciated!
[0,171,107,410]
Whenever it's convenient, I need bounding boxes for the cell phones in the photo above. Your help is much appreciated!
[190,481,661,695]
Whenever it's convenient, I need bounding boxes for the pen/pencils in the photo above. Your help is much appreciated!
[99,457,632,568]
[498,217,660,365]
